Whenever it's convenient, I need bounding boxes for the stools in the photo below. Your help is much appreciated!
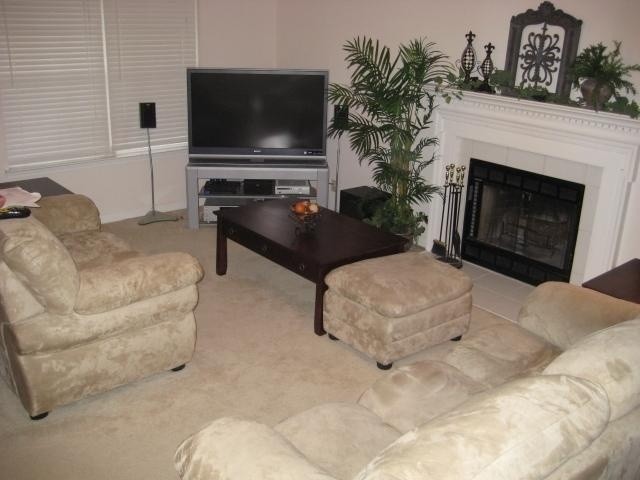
[323,251,472,370]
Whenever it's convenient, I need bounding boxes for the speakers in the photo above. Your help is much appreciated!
[334,105,348,126]
[140,103,156,128]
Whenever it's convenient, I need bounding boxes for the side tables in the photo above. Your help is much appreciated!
[581,257,640,304]
[0,177,74,196]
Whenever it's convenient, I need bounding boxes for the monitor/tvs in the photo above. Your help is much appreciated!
[187,68,329,167]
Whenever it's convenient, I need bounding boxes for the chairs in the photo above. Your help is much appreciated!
[0,193,204,421]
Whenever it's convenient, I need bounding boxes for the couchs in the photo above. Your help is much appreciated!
[173,282,640,480]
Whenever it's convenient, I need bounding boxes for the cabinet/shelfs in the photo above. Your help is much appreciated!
[185,163,329,230]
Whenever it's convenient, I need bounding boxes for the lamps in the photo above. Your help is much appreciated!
[461,30,475,84]
[477,43,495,93]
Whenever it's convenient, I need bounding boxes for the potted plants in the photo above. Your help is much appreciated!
[565,39,640,114]
[323,36,456,243]
